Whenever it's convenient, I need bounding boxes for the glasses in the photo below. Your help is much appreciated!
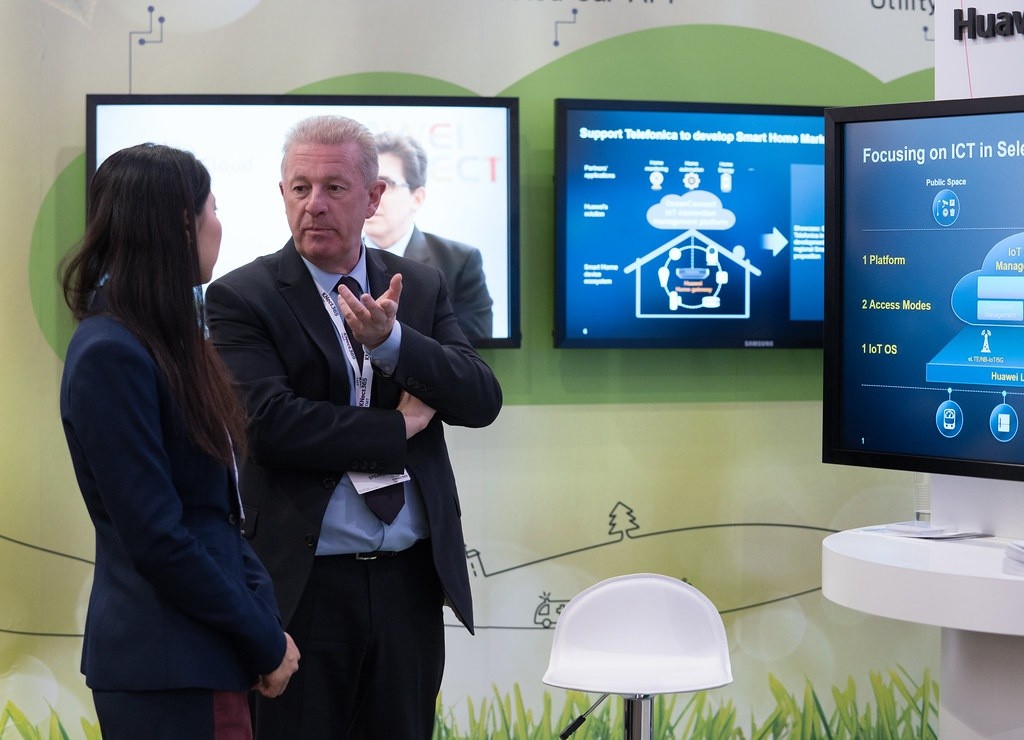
[378,174,410,192]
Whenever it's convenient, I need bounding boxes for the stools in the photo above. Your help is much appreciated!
[541,572,733,740]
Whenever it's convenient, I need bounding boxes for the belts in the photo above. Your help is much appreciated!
[317,544,433,561]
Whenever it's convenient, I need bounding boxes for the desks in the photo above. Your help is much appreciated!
[822,519,1024,739]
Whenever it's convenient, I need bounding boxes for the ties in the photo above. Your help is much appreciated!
[331,275,405,526]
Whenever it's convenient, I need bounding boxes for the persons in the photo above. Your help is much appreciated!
[62,141,302,740]
[360,131,493,339]
[200,113,504,740]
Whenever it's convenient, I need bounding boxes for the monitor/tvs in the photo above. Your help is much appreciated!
[553,99,828,351]
[85,93,520,349]
[820,95,1023,482]
